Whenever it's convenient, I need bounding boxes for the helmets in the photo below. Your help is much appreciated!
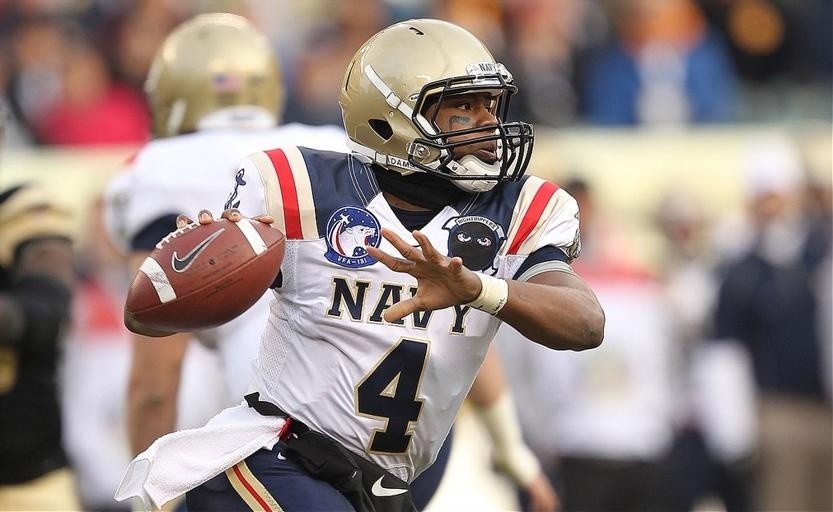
[338,17,513,179]
[0,183,76,243]
[144,10,284,139]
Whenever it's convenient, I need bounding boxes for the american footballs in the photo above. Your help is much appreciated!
[128,218,285,332]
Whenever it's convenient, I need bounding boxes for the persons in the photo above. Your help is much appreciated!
[0,185,138,512]
[489,140,833,512]
[0,0,739,146]
